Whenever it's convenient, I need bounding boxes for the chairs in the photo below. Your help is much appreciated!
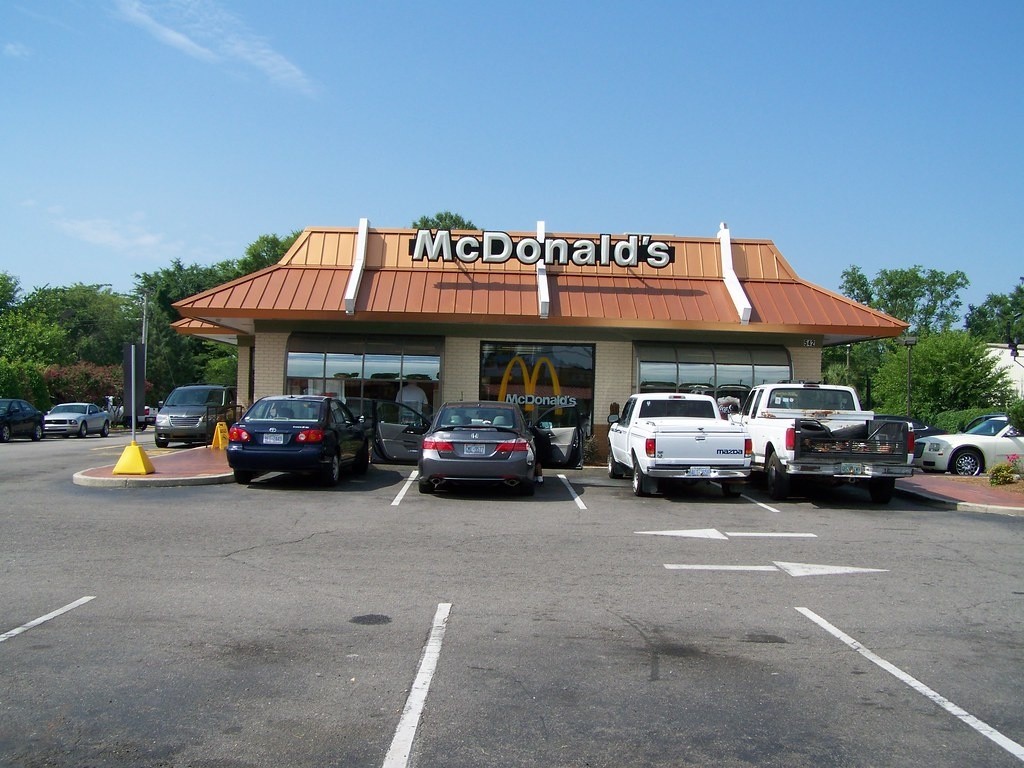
[493,416,504,426]
[450,416,463,424]
[271,409,295,418]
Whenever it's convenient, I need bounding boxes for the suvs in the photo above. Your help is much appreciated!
[154,382,237,448]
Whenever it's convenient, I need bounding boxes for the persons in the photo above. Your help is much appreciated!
[395,376,428,425]
[529,425,551,486]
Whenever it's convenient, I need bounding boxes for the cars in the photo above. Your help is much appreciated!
[225,394,370,486]
[0,398,45,442]
[874,413,949,457]
[366,398,584,496]
[912,416,1024,476]
[956,414,1006,435]
[41,402,112,439]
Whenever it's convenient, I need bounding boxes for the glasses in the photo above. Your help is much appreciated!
[1016,431,1019,432]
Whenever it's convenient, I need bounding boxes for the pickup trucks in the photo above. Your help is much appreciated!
[607,392,753,497]
[93,395,159,431]
[726,381,917,505]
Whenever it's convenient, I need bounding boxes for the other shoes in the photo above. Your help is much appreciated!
[536,475,544,487]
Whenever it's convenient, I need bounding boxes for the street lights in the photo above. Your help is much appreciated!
[897,336,917,416]
